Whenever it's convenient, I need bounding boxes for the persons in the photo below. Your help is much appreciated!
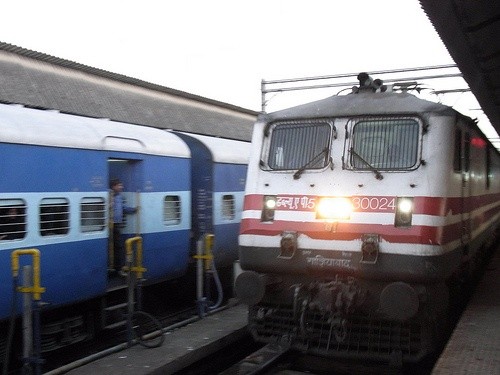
[109,179,139,277]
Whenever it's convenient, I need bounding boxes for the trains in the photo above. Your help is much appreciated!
[233,72,500,364]
[0,102,284,362]
